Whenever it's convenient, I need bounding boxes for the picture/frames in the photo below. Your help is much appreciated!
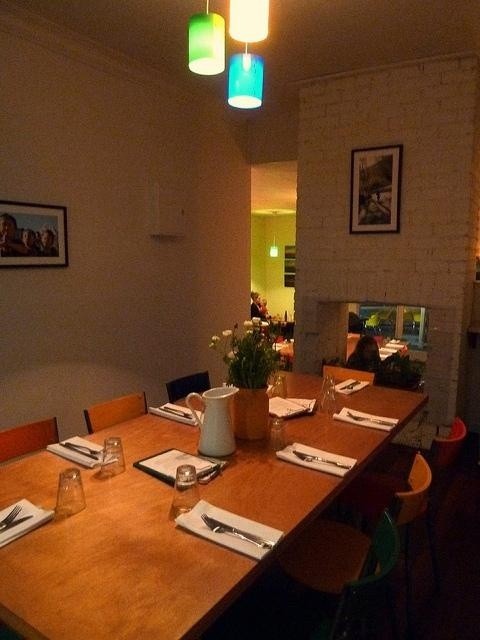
[0,200,70,270]
[349,144,403,235]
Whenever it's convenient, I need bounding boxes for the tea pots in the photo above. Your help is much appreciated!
[186,386,240,458]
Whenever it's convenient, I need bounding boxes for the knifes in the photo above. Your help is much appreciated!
[347,415,397,426]
[0,515,33,535]
[340,381,358,390]
[293,451,352,469]
[211,519,272,549]
[60,444,99,460]
[159,407,192,419]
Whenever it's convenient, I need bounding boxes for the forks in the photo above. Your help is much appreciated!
[0,505,22,528]
[347,412,393,427]
[164,407,191,417]
[65,442,103,454]
[347,382,360,390]
[201,514,268,548]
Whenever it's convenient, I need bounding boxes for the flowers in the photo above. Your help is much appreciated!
[208,316,294,390]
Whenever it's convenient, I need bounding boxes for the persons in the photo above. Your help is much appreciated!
[345,335,388,381]
[257,299,268,315]
[0,212,60,258]
[251,291,269,323]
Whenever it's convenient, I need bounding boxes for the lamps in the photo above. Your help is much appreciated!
[187,0,264,112]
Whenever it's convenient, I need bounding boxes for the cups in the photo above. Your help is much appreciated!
[272,375,286,399]
[269,418,287,452]
[100,437,125,476]
[56,467,86,517]
[172,464,199,513]
